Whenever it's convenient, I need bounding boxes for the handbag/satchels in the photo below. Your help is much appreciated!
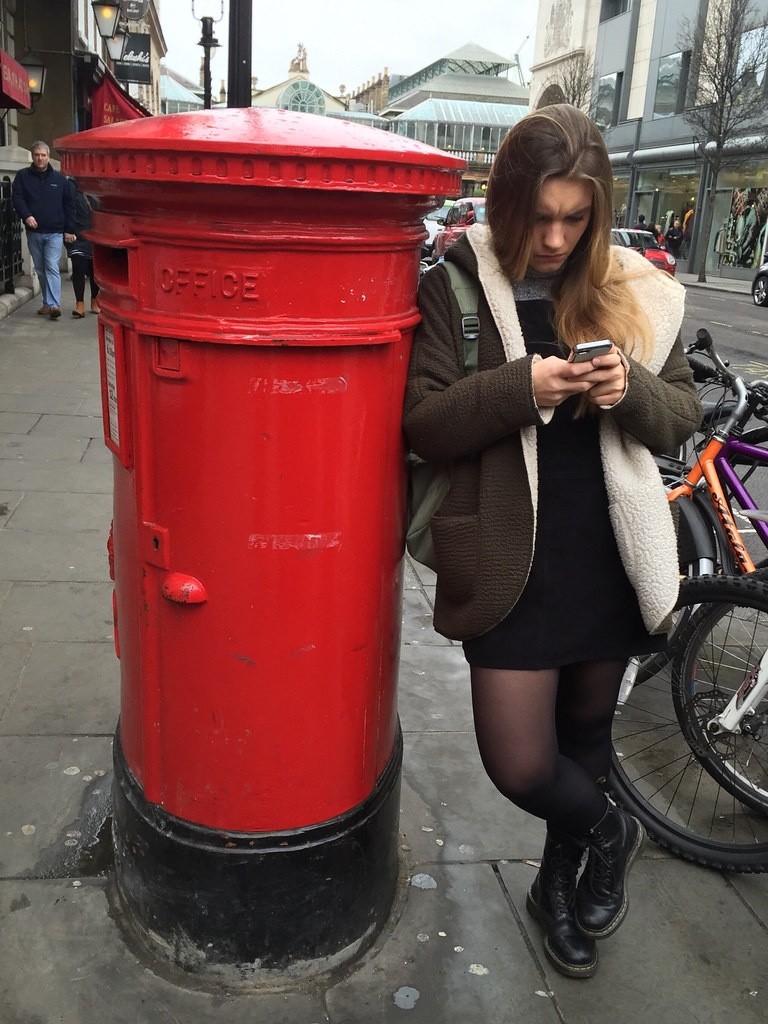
[405,259,482,573]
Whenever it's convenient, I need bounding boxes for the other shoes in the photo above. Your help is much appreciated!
[38,306,49,313]
[50,307,62,318]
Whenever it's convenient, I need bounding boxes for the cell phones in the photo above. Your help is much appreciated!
[568,340,612,365]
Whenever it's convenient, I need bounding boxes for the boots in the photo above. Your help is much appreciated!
[91,299,100,314]
[526,824,600,978]
[72,301,85,317]
[573,793,644,940]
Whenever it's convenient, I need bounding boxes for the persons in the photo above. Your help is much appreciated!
[662,209,675,237]
[619,204,627,228]
[62,188,105,317]
[13,141,76,321]
[632,215,683,254]
[682,208,695,242]
[401,107,705,973]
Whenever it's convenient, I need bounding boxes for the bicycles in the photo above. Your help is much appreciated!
[601,327,768,875]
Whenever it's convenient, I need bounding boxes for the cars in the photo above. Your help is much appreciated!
[430,198,486,263]
[422,200,457,259]
[751,251,768,307]
[611,227,677,276]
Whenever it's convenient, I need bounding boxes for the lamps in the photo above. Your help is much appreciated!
[15,46,47,115]
[90,0,121,39]
